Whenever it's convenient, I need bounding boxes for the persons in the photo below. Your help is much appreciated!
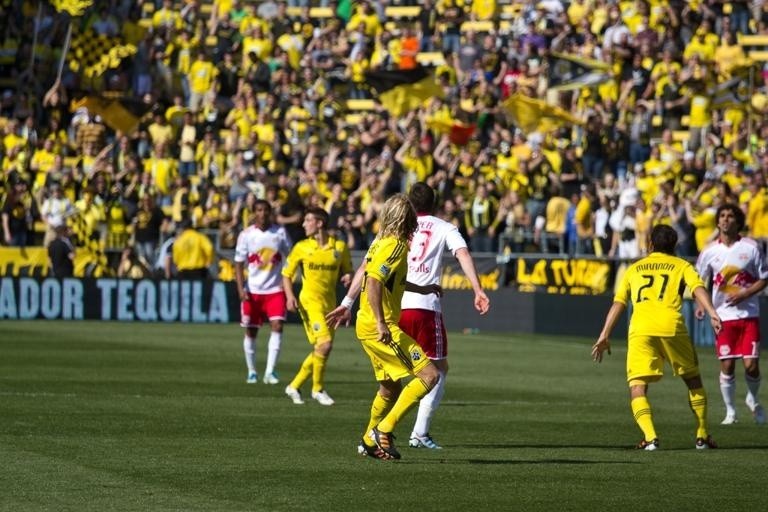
[40,186,71,242]
[3,183,39,243]
[132,194,166,260]
[78,190,106,241]
[48,227,75,280]
[281,210,355,406]
[690,203,766,426]
[324,183,492,448]
[109,185,133,252]
[235,203,294,385]
[174,220,215,314]
[442,182,685,254]
[687,181,768,250]
[591,224,722,451]
[155,235,172,279]
[165,183,326,203]
[166,202,235,222]
[1,1,766,180]
[323,193,439,460]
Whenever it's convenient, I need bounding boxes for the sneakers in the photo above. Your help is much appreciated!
[358,438,394,459]
[695,434,719,450]
[246,370,259,384]
[409,432,443,449]
[312,389,335,405]
[745,394,767,425]
[262,372,280,385]
[369,423,401,459]
[719,412,739,425]
[633,439,659,450]
[285,384,305,405]
